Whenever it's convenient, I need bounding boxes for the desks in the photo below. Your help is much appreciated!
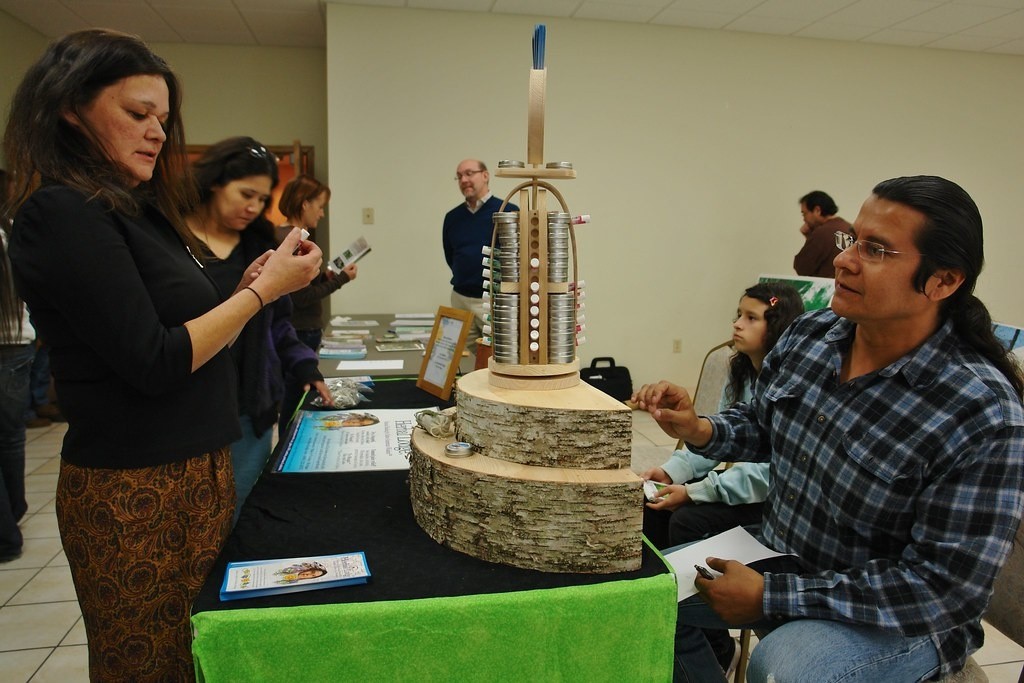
[315,313,436,377]
[191,377,678,682]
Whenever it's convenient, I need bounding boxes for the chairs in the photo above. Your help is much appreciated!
[937,509,1024,683]
[629,340,738,475]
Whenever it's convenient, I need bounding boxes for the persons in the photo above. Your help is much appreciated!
[283,562,327,583]
[795,192,852,245]
[631,175,1024,683]
[442,160,520,355]
[636,284,805,552]
[273,175,356,440]
[0,28,323,683]
[175,136,332,534]
[324,413,379,428]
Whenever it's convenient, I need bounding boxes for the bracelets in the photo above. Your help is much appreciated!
[244,286,264,308]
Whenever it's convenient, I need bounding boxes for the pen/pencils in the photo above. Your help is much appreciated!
[694,564,714,580]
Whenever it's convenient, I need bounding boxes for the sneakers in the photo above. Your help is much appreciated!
[720,637,741,679]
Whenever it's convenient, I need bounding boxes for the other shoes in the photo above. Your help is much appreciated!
[1,546,21,563]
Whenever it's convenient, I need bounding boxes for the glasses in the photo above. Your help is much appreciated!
[454,171,483,180]
[248,146,271,158]
[834,231,924,262]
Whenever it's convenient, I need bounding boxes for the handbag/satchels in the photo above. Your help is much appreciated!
[580,357,633,401]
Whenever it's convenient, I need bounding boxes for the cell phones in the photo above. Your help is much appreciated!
[694,564,714,581]
